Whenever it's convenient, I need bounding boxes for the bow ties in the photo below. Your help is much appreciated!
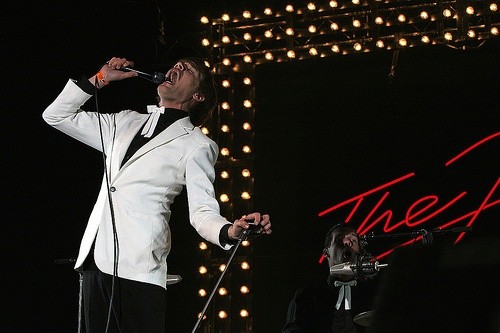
[140,105,166,138]
[334,280,357,310]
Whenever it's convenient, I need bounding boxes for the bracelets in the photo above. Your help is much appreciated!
[96,71,110,86]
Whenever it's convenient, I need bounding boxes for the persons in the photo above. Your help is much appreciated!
[281,220,385,333]
[41,56,273,333]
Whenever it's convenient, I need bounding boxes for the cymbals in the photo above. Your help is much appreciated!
[353,310,377,328]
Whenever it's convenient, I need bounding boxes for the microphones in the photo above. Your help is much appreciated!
[119,66,165,84]
[330,261,379,277]
